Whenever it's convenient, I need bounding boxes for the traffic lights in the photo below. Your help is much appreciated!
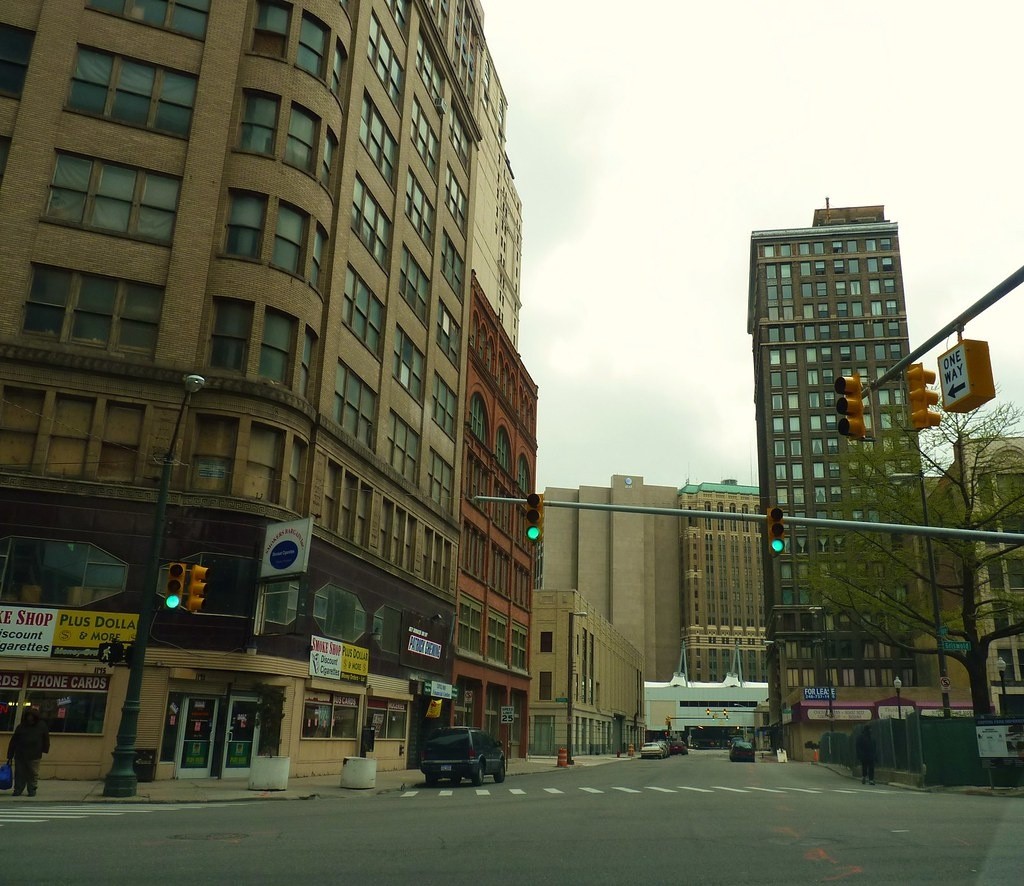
[906,362,941,431]
[766,507,786,557]
[566,612,588,764]
[834,372,867,442]
[163,562,187,612]
[527,493,544,544]
[186,564,210,612]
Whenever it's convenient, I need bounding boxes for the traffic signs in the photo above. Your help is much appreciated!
[939,626,948,635]
[942,642,971,650]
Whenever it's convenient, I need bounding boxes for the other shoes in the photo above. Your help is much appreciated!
[28,793,35,797]
[861,779,866,784]
[869,780,875,785]
[12,792,21,796]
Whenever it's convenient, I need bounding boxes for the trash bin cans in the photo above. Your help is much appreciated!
[134,746,158,782]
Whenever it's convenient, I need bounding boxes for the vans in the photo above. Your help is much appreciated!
[420,726,506,785]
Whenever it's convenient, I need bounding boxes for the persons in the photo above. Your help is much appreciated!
[6,707,51,797]
[855,725,877,784]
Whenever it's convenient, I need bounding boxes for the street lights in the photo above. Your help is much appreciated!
[996,657,1007,718]
[101,375,206,797]
[894,676,902,719]
[808,605,834,732]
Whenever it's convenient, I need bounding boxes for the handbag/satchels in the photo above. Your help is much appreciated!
[0,759,12,790]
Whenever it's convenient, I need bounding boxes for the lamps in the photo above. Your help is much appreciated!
[244,618,259,656]
[370,625,382,640]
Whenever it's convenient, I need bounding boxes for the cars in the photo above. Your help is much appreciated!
[730,741,756,762]
[669,741,688,755]
[641,742,662,760]
